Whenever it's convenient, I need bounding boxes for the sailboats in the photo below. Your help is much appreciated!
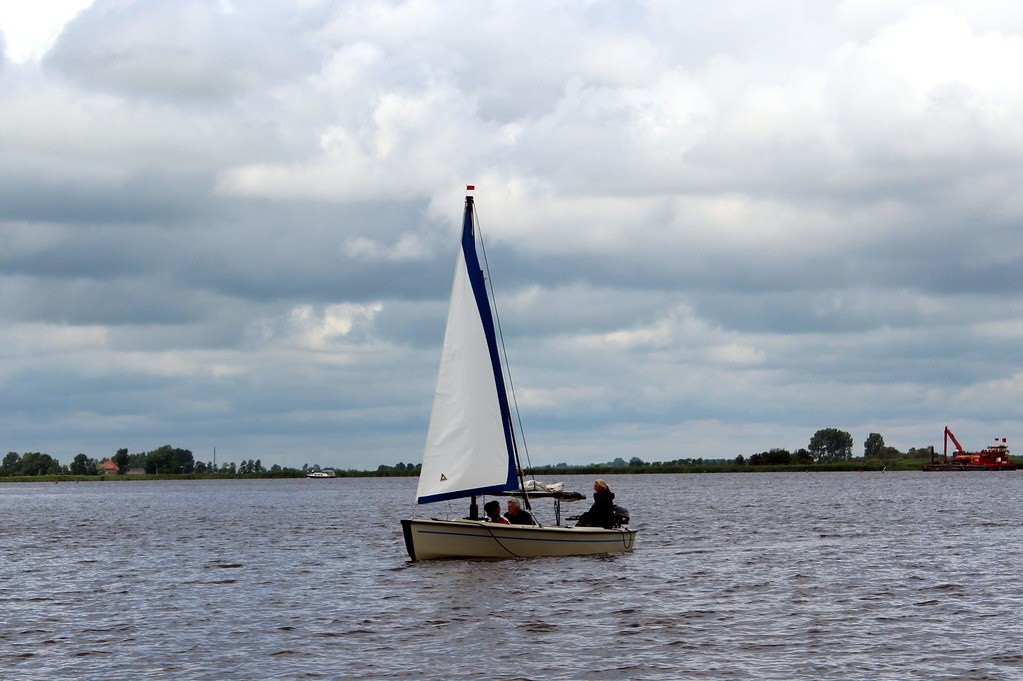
[399,185,638,564]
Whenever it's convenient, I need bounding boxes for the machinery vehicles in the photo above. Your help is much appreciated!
[941,426,1010,465]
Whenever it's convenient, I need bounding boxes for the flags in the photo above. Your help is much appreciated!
[995,438,999,441]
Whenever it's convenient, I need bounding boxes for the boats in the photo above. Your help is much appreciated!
[306,470,336,478]
[923,447,944,471]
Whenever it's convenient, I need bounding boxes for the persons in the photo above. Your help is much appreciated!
[575,479,615,529]
[485,501,511,524]
[504,500,535,525]
